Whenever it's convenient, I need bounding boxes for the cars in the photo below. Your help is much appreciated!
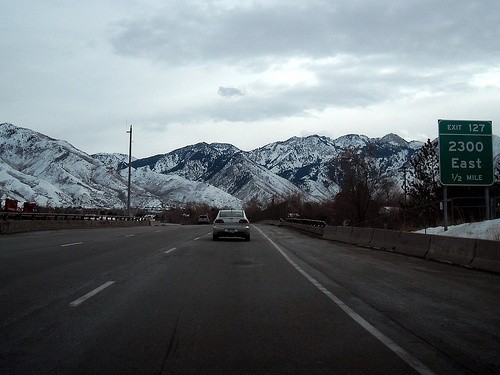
[197,215,210,225]
[213,209,251,241]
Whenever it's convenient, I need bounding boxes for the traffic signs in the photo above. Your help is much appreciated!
[438,118,495,186]
